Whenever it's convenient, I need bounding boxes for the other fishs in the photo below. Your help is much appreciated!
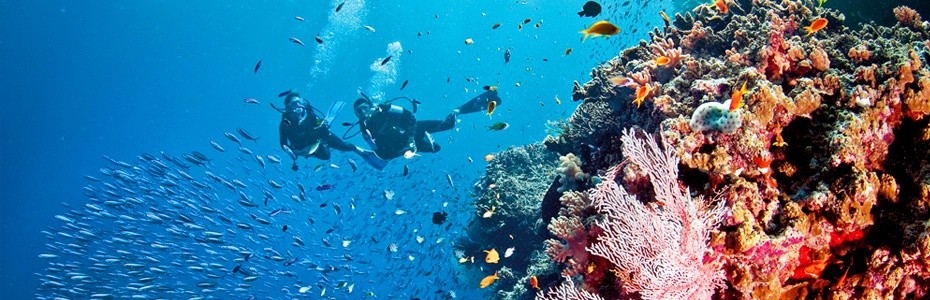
[711,0,728,12]
[32,0,699,300]
[689,80,747,135]
[803,18,828,37]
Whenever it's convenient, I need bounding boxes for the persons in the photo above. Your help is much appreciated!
[354,89,506,160]
[279,91,387,171]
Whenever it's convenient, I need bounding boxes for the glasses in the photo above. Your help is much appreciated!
[357,104,371,114]
[288,99,304,108]
